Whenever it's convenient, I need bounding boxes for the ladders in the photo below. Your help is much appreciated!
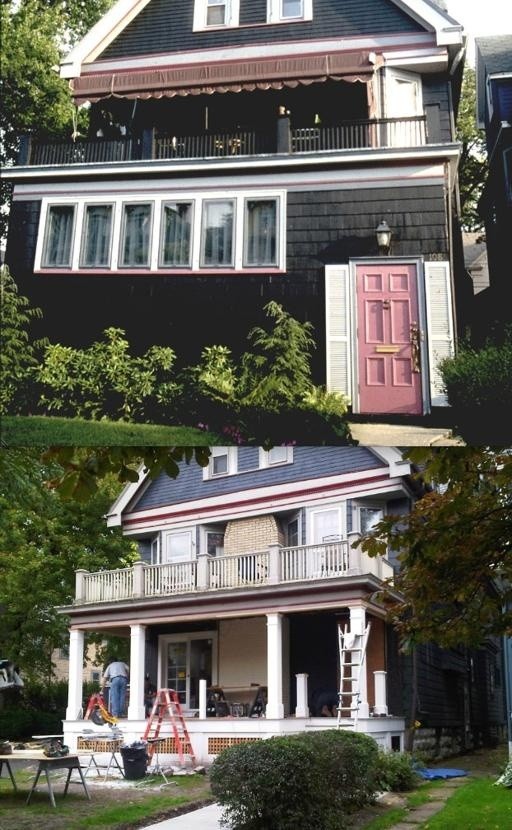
[84,692,116,726]
[144,688,197,772]
[336,621,371,733]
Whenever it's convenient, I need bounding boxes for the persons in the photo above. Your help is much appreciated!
[101,657,129,719]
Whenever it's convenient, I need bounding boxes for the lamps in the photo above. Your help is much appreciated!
[375,219,393,252]
[111,723,119,740]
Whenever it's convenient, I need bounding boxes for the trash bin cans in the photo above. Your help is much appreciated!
[120,741,149,780]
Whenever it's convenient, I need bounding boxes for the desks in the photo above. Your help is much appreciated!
[0,752,92,809]
